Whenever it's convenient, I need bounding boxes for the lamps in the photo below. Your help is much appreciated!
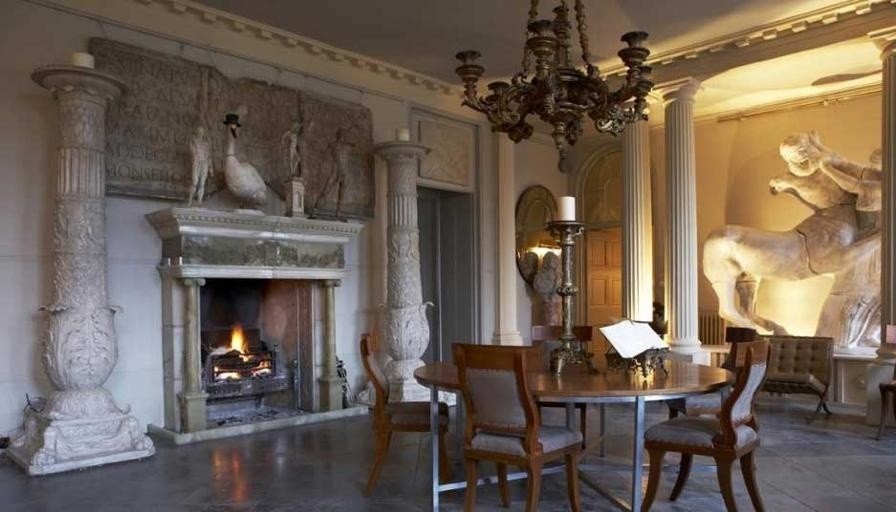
[455,0,654,175]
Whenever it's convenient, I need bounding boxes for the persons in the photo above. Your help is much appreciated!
[310,126,356,218]
[280,120,301,180]
[768,129,882,356]
[188,125,214,208]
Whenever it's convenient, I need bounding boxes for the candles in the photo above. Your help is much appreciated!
[556,195,576,222]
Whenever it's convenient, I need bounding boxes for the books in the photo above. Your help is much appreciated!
[599,318,672,359]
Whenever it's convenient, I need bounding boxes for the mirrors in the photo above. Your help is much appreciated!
[513,184,563,295]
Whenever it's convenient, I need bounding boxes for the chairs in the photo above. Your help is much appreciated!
[639,340,771,511]
[447,340,585,511]
[665,325,765,490]
[528,321,609,457]
[359,331,459,500]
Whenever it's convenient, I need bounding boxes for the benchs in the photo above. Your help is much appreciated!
[719,334,836,417]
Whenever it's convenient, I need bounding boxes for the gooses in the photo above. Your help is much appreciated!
[224,123,268,209]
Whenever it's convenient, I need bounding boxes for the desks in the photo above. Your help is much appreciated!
[409,362,738,510]
